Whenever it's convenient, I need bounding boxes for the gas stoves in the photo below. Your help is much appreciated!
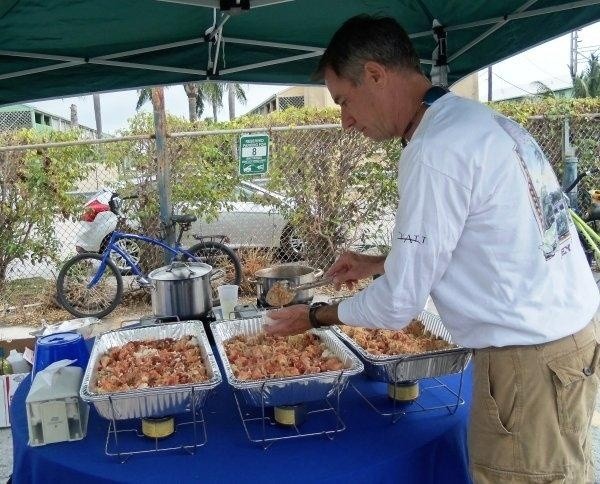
[229,295,332,320]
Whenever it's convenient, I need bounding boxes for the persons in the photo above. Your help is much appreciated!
[261,11,599,483]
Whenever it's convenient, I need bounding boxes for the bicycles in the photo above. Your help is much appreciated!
[56,192,242,319]
[564,167,600,270]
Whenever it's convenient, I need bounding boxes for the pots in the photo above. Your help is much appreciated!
[145,260,213,318]
[246,263,325,305]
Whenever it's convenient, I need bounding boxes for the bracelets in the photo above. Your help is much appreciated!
[308,301,332,329]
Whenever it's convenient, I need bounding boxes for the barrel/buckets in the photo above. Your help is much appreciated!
[31,331,90,390]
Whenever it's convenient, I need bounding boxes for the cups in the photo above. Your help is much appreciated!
[217,284,239,321]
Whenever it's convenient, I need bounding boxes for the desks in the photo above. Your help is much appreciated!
[8,323,473,484]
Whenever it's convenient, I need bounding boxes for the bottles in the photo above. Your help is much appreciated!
[570,205,590,220]
[0,348,14,375]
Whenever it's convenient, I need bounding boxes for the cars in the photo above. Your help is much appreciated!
[72,172,370,259]
